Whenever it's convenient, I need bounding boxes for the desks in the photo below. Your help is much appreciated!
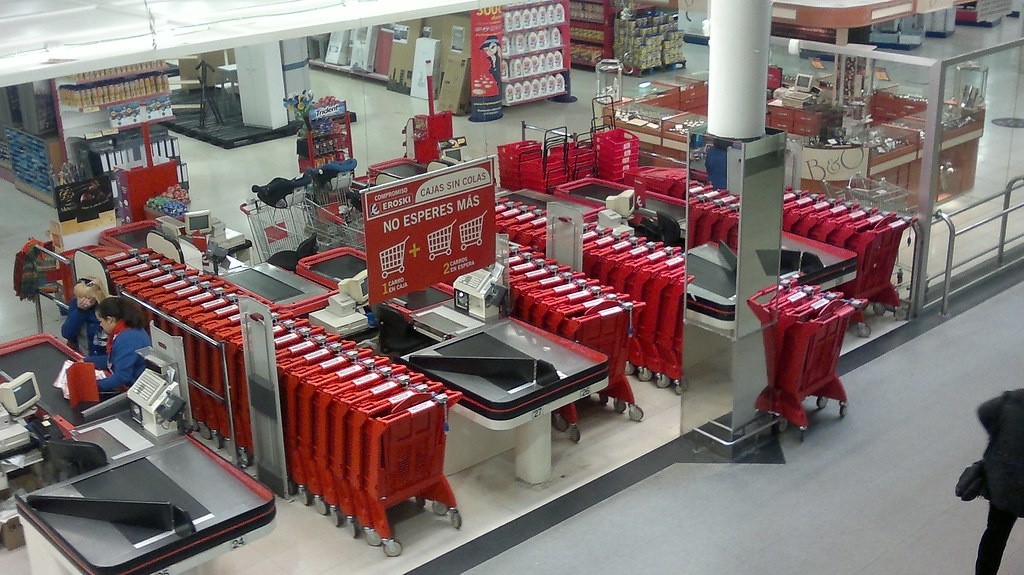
[217,64,238,118]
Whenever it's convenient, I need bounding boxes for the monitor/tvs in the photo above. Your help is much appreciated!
[184,209,213,239]
[794,73,816,93]
[337,268,369,305]
[439,147,465,164]
[605,189,637,222]
[0,370,42,421]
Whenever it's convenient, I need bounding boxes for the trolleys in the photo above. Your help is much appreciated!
[50,230,463,559]
[241,94,919,446]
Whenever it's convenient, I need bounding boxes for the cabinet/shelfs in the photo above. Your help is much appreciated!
[640,82,707,117]
[499,0,687,107]
[766,100,846,135]
[53,59,180,166]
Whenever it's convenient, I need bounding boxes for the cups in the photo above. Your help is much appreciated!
[139,102,147,122]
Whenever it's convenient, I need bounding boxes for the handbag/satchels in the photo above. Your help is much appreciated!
[956,463,983,503]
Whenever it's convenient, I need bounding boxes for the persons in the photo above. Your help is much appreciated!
[60,276,109,355]
[78,296,152,392]
[975,388,1024,575]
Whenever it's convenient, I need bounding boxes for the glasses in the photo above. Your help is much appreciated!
[77,278,93,287]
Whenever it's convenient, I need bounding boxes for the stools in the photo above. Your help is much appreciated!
[215,82,240,118]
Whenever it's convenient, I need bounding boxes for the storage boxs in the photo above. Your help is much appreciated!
[436,53,471,116]
[410,38,443,99]
[385,19,425,95]
[325,26,378,73]
